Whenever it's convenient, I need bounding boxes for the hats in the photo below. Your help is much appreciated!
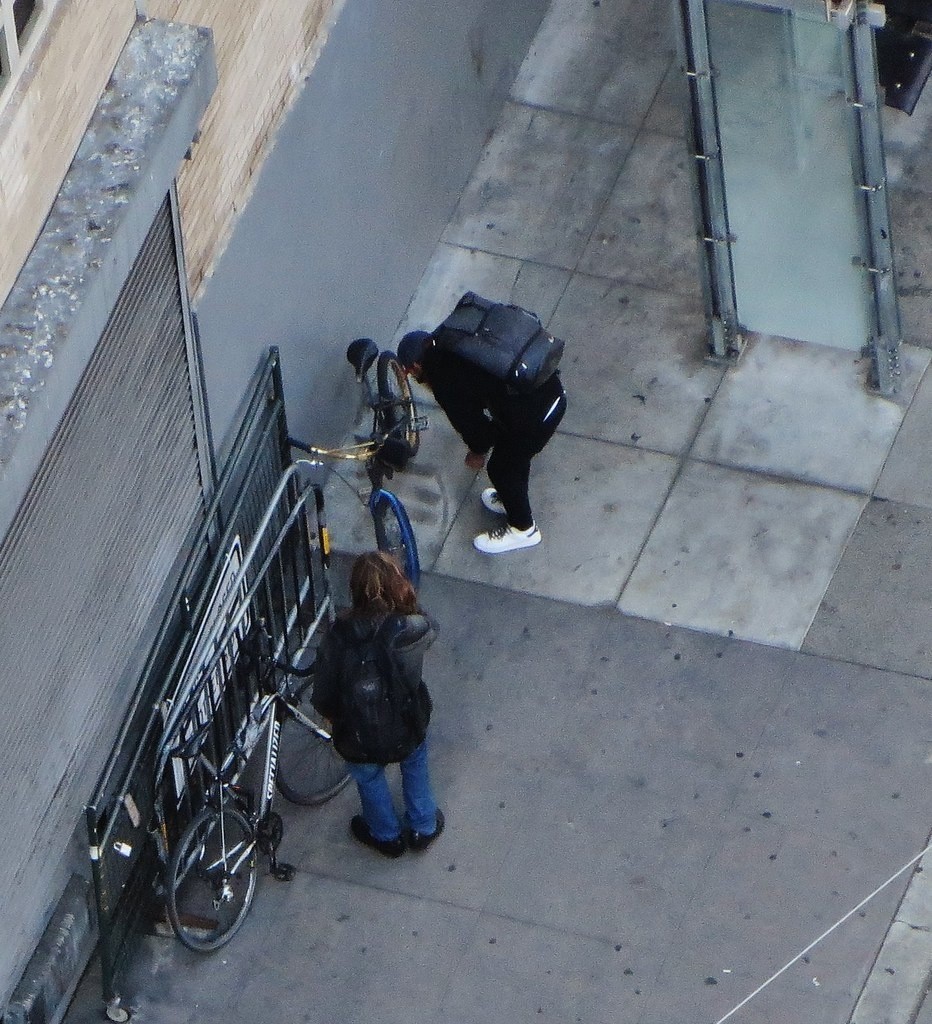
[396,331,432,363]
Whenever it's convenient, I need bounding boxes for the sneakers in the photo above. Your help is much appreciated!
[473,514,541,553]
[481,487,506,515]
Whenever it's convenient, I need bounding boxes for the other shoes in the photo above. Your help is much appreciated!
[351,815,405,859]
[408,805,445,852]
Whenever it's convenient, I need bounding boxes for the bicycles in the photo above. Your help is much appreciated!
[288,338,428,605]
[168,617,355,954]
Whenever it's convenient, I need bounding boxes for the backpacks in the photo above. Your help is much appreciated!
[330,616,422,764]
[426,291,565,392]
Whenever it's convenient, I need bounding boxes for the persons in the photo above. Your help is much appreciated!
[310,548,444,857]
[397,326,566,552]
